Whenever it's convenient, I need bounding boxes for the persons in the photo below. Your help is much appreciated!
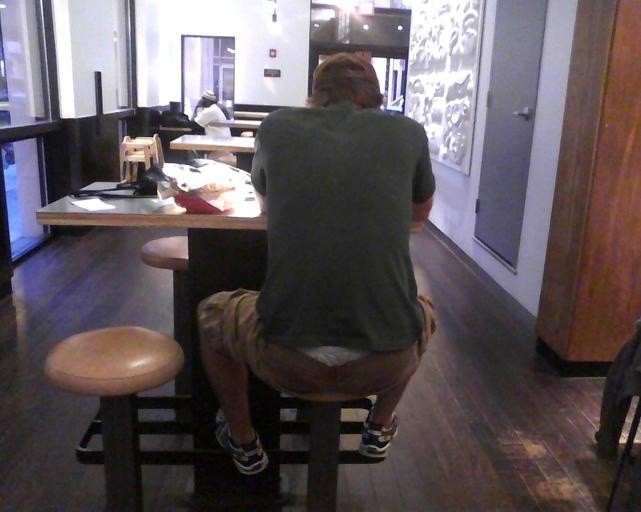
[195,51,441,478]
[183,90,232,164]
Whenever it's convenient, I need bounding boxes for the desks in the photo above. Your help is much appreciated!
[36,111,424,511]
[118,109,270,182]
[36,182,424,509]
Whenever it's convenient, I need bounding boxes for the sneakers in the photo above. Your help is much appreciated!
[358,404,399,458]
[215,408,269,475]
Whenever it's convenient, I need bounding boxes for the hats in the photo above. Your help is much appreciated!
[202,89,216,102]
[313,52,378,85]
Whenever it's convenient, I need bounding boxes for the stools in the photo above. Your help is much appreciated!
[270,387,372,510]
[141,236,190,417]
[42,324,185,511]
